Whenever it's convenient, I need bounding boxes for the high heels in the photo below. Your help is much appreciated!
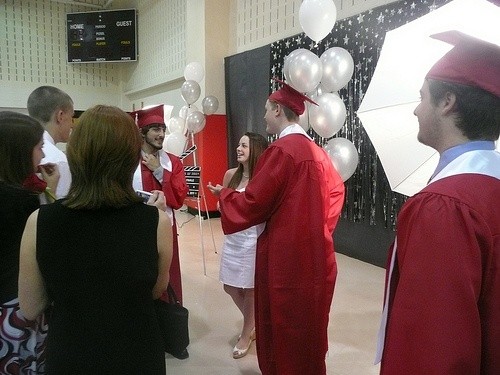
[232,336,253,359]
[238,326,256,341]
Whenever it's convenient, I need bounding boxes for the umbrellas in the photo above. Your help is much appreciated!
[355,0,500,197]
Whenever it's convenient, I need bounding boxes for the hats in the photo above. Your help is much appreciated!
[427,30,500,98]
[129,103,166,127]
[266,76,319,126]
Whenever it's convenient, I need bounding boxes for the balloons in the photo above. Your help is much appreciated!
[284,48,358,182]
[167,62,219,136]
[298,0,336,42]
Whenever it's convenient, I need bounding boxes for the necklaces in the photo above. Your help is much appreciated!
[243,172,249,178]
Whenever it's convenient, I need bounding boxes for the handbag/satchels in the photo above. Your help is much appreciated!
[152,283,190,359]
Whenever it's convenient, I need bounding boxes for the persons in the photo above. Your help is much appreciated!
[207,78,344,375]
[376,30,500,375]
[0,86,189,375]
